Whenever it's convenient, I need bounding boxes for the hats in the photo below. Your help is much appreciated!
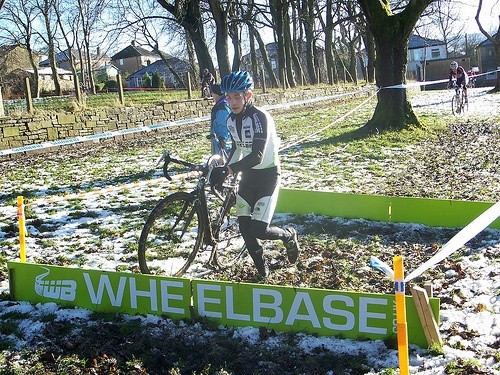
[211,83,223,96]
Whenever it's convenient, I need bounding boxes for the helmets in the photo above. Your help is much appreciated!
[202,69,208,75]
[220,70,256,91]
[450,60,459,69]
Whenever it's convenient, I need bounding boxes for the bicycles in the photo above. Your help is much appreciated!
[470,76,477,89]
[201,80,212,97]
[207,133,236,167]
[138,150,249,276]
[447,81,469,116]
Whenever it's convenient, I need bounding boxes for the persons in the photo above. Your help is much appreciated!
[198,67,301,281]
[446,61,478,113]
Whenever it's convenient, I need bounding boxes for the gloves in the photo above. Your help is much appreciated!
[209,167,229,188]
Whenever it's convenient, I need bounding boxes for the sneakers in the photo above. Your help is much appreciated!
[283,227,300,265]
[248,271,269,284]
